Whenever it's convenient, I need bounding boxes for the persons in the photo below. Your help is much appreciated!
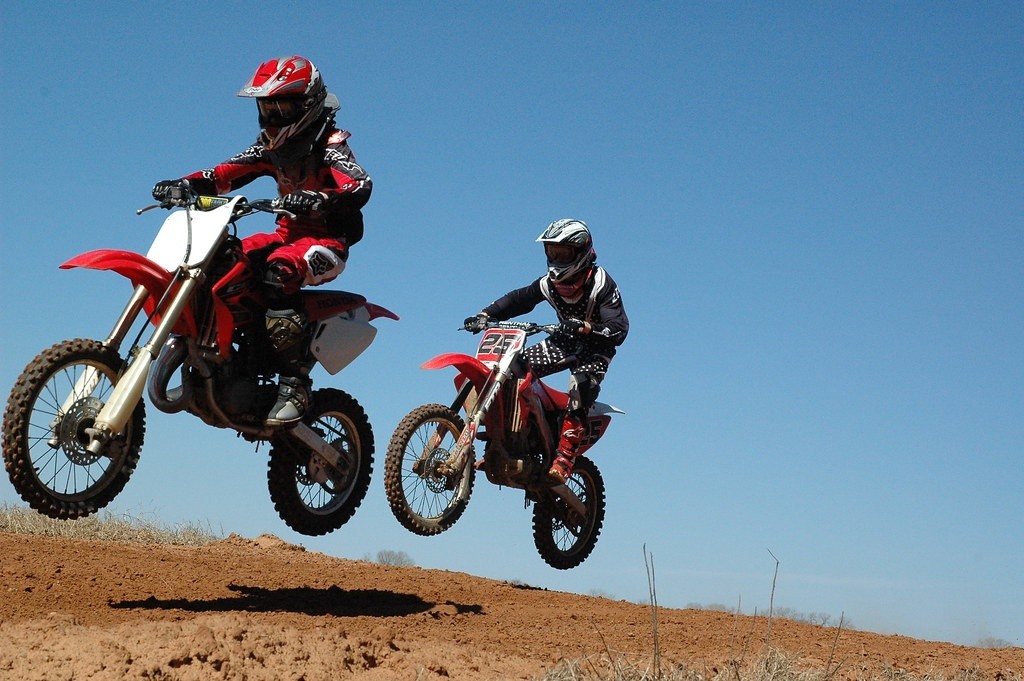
[151,55,373,427]
[463,219,630,486]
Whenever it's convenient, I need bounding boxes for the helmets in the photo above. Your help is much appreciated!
[237,55,324,151]
[535,218,596,283]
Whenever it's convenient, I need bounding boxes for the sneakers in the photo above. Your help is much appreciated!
[266,361,315,426]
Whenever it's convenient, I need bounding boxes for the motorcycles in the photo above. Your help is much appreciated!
[2,181,400,537]
[384,314,625,570]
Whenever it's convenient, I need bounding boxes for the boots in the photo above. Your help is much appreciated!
[549,419,585,483]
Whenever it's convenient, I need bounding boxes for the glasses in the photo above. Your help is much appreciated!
[546,238,592,264]
[256,86,328,128]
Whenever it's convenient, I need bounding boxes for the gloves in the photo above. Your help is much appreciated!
[152,178,185,203]
[282,190,329,221]
[464,312,490,335]
[559,318,591,337]
